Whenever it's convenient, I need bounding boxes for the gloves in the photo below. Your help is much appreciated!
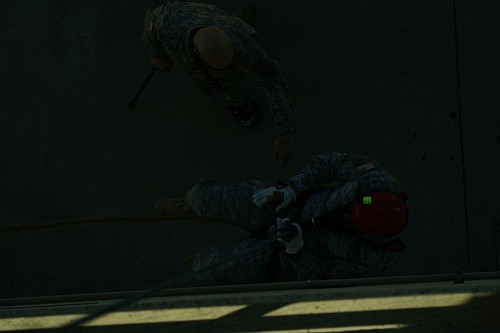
[149,55,173,72]
[273,136,295,163]
[252,184,296,215]
[276,218,304,255]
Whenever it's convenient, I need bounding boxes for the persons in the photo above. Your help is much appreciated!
[151,149,411,285]
[141,1,298,171]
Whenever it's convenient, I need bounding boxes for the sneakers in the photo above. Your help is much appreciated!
[154,198,189,217]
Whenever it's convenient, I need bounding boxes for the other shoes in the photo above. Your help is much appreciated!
[239,2,257,21]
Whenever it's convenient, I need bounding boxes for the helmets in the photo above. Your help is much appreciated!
[349,189,410,239]
